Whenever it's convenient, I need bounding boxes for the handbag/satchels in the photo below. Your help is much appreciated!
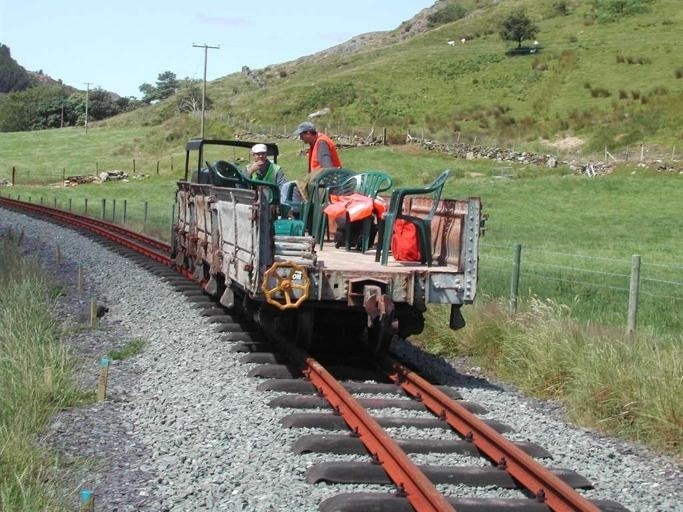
[391,215,422,262]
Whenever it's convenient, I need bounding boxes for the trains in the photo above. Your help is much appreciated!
[168,136,489,357]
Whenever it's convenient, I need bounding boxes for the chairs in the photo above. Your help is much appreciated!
[213,159,449,269]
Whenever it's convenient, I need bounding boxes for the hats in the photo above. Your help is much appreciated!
[252,144,268,154]
[293,122,316,136]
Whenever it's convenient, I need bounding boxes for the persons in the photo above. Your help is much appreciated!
[293,120,341,236]
[237,143,290,225]
